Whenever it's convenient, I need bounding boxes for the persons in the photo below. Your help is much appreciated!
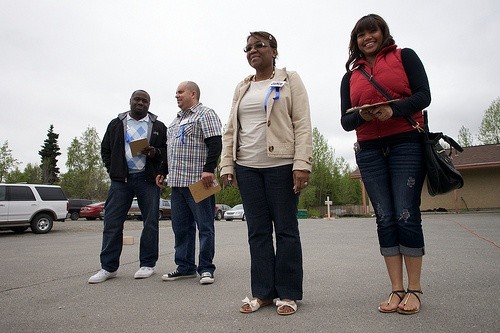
[156,80,222,285]
[340,14,431,314]
[218,32,313,316]
[88,90,169,284]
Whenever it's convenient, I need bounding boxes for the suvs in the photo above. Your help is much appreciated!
[0,183,69,234]
[67,199,95,220]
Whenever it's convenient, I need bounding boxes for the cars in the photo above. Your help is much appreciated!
[224,204,247,221]
[214,203,232,220]
[80,202,106,220]
[99,198,172,221]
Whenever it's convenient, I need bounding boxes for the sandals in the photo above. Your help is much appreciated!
[378,290,406,313]
[276,300,298,314]
[240,296,273,313]
[397,288,423,314]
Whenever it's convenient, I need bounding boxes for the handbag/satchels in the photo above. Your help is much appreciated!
[420,109,463,196]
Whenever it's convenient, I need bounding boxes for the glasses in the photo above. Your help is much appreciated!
[242,41,273,53]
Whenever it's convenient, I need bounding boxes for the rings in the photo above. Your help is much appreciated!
[377,111,382,115]
[305,181,308,186]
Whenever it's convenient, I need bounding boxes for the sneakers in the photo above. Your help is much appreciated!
[88,269,117,284]
[134,266,156,278]
[161,268,197,281]
[200,271,215,284]
